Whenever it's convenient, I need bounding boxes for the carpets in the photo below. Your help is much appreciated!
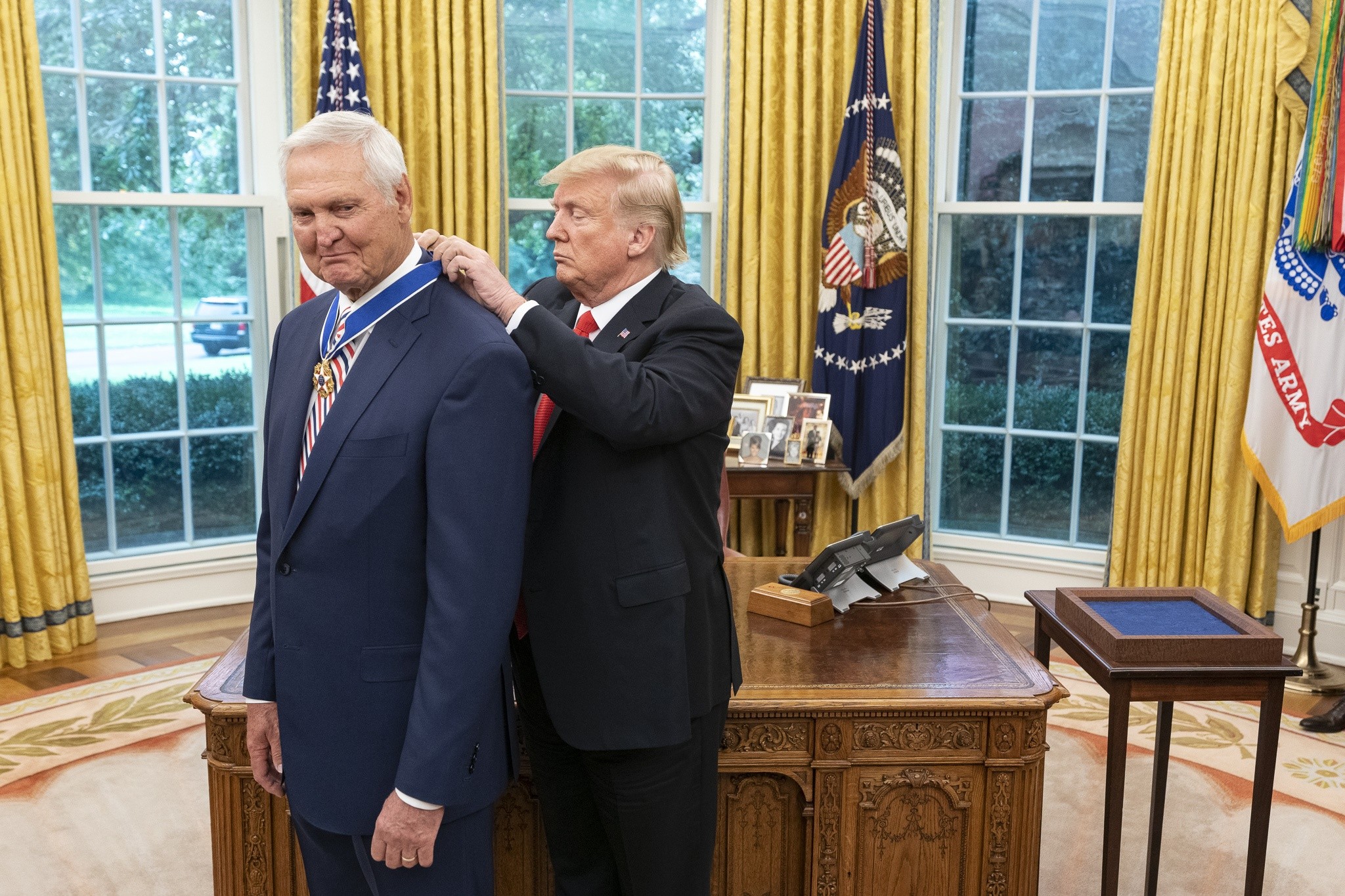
[1032,650,1345,896]
[0,653,222,896]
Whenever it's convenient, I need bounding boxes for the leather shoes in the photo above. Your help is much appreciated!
[1299,699,1345,733]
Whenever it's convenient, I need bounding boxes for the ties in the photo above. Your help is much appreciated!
[296,308,359,494]
[514,311,599,639]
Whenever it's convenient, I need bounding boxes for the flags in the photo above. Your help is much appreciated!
[1240,0,1345,544]
[298,0,375,304]
[810,0,908,502]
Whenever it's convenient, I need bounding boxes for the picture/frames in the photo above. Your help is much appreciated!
[800,417,833,464]
[743,376,806,415]
[783,392,831,438]
[739,429,771,467]
[763,415,796,458]
[728,393,775,450]
[783,438,802,465]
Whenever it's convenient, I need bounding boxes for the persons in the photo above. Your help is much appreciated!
[743,435,764,464]
[412,143,745,896]
[242,111,536,896]
[732,413,757,437]
[786,441,800,463]
[788,395,823,439]
[806,425,822,459]
[765,418,790,458]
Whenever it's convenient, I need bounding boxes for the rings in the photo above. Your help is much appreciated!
[401,854,417,862]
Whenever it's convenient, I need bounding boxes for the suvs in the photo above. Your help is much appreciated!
[191,296,250,354]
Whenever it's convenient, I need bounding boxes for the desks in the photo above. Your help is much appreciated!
[1025,588,1303,896]
[726,453,850,557]
[185,554,1071,896]
